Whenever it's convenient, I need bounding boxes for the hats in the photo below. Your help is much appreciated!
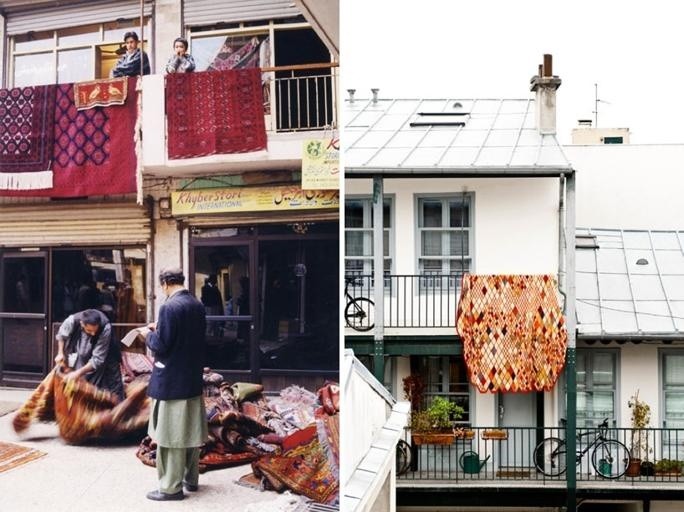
[160,265,185,281]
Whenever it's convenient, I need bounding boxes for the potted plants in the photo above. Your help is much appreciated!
[623,389,650,476]
[652,459,684,476]
[409,395,465,444]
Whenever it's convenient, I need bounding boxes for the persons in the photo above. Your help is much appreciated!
[120,268,209,500]
[110,30,150,77]
[164,37,196,75]
[53,309,124,398]
[12,266,262,341]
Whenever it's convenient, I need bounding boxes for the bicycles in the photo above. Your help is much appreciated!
[396,440,412,476]
[343,274,374,332]
[533,416,631,479]
[397,445,406,473]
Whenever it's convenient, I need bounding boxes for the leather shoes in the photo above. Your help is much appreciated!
[147,490,184,501]
[182,481,197,491]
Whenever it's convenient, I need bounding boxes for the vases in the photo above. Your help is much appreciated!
[641,460,653,477]
